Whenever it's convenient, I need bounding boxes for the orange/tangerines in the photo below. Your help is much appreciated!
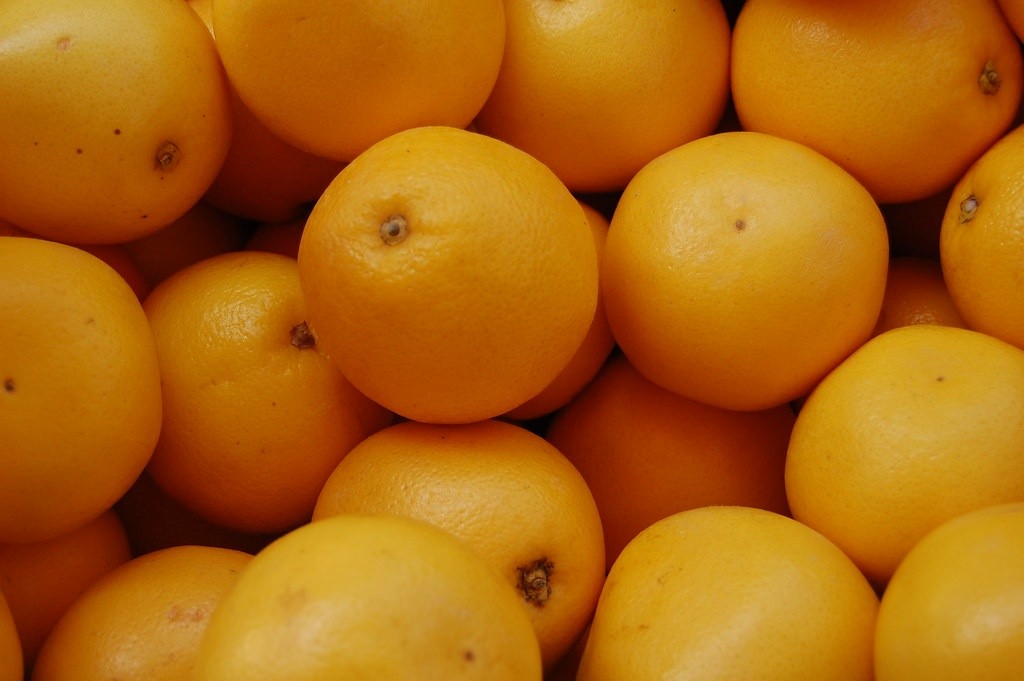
[0,0,1024,681]
